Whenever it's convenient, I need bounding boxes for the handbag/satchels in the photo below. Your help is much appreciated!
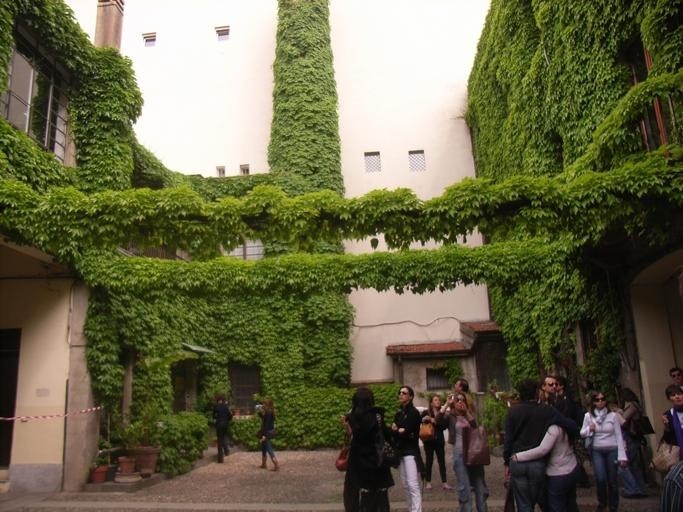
[419,409,435,441]
[584,436,594,449]
[465,423,491,466]
[647,441,682,474]
[382,432,404,469]
[255,430,264,439]
[334,446,352,473]
[632,415,656,435]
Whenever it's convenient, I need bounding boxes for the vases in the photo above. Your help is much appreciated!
[117,457,135,474]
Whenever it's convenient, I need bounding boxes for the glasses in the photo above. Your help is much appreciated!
[398,391,408,396]
[672,373,681,378]
[547,382,559,387]
[595,398,606,402]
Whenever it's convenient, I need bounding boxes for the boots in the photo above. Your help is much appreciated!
[270,456,280,471]
[258,455,267,469]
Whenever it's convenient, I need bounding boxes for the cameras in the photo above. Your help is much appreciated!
[452,395,462,400]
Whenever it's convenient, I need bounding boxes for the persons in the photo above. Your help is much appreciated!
[254,397,280,471]
[212,393,236,463]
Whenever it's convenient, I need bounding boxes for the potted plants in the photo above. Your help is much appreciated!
[87,453,107,484]
[123,417,164,476]
